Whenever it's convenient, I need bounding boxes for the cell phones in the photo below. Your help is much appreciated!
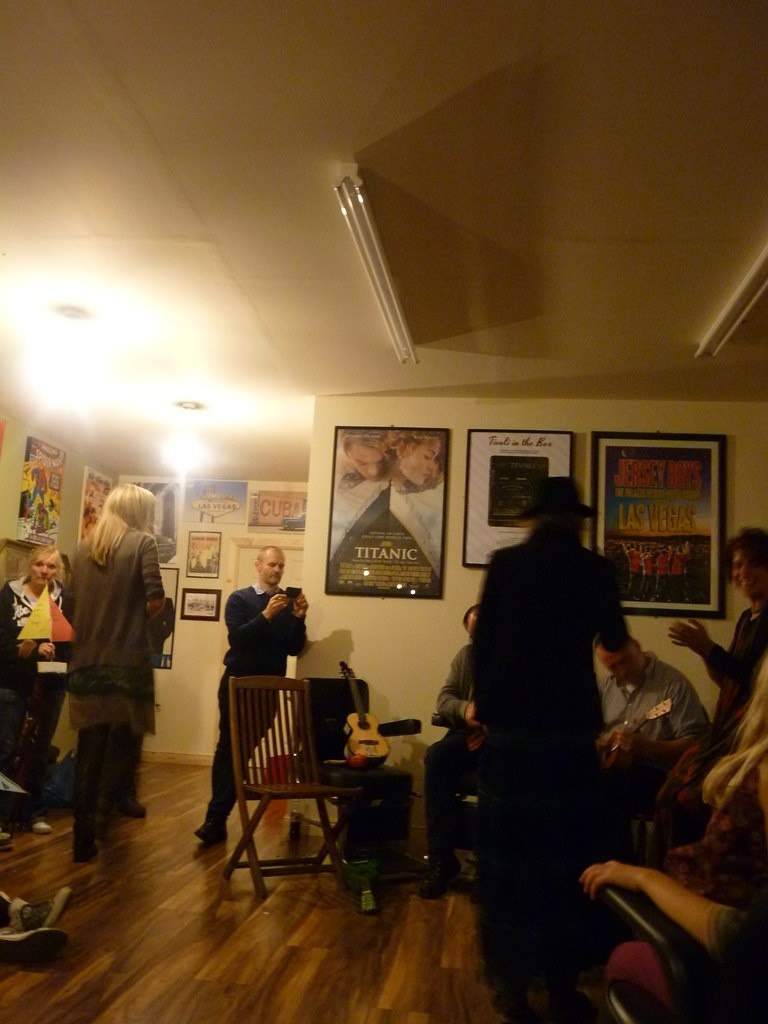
[286,587,302,598]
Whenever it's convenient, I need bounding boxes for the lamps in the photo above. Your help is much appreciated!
[694,243,768,360]
[332,161,428,366]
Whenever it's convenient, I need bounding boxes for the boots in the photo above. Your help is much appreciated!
[97,763,124,840]
[73,764,102,862]
[9,886,72,934]
[0,927,68,962]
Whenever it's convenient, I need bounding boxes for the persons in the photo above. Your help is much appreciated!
[607,541,704,602]
[335,428,448,495]
[467,475,629,1024]
[81,506,98,539]
[66,483,166,862]
[595,637,707,857]
[580,651,768,1024]
[0,887,75,965]
[193,545,308,842]
[415,604,480,900]
[0,543,75,850]
[667,524,768,735]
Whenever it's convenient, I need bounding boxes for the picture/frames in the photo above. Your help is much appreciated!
[180,588,221,622]
[76,465,113,556]
[183,478,248,524]
[590,432,731,620]
[244,490,308,535]
[17,436,65,549]
[127,482,182,564]
[323,426,451,599]
[463,429,574,567]
[185,531,222,579]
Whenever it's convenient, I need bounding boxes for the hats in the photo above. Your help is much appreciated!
[519,477,593,520]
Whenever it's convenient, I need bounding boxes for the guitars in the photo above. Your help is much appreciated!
[339,660,390,766]
[599,698,672,771]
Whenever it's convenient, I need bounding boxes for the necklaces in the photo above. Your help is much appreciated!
[750,607,762,613]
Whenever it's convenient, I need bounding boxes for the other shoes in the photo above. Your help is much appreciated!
[471,874,479,902]
[119,791,146,818]
[420,851,460,898]
[195,818,226,843]
[23,820,51,834]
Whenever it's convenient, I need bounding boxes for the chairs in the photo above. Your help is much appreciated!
[585,858,768,1024]
[228,675,350,895]
[294,678,424,885]
